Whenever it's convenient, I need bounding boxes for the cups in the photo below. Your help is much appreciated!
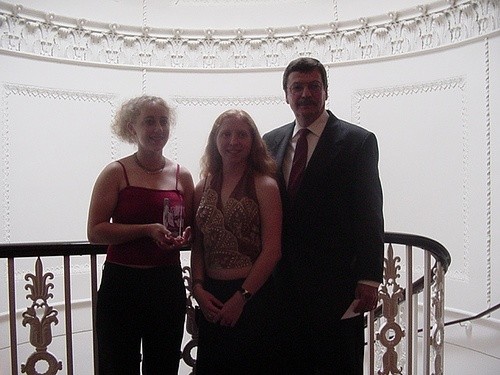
[163,198,182,239]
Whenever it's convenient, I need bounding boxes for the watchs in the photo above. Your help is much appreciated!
[238,287,253,300]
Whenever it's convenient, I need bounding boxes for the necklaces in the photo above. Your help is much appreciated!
[132,150,167,175]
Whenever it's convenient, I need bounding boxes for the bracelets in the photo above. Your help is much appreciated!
[191,278,204,286]
[187,231,193,242]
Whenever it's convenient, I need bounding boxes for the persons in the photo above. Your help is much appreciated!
[183,107,289,375]
[85,96,196,375]
[259,57,385,375]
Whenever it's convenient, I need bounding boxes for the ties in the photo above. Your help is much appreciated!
[288,129,311,194]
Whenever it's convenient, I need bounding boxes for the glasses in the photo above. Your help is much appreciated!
[286,83,325,96]
